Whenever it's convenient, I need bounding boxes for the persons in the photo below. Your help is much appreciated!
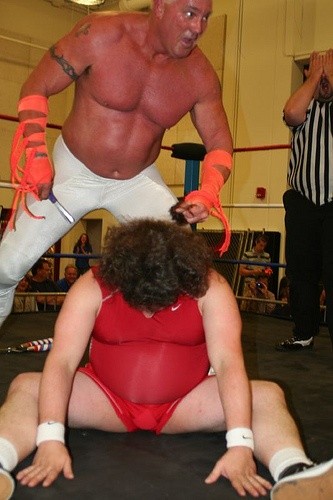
[15,273,39,292]
[73,233,93,274]
[57,265,78,292]
[40,245,54,275]
[0,0,235,328]
[273,49,333,351]
[239,233,273,303]
[273,269,325,317]
[0,220,333,500]
[30,259,61,292]
[240,275,275,313]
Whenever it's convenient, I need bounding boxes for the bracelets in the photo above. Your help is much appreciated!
[225,427,255,450]
[36,421,65,445]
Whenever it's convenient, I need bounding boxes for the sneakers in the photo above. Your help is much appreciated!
[275,335,314,351]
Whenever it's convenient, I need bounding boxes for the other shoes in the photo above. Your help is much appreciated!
[0,463,15,500]
[269,462,333,500]
